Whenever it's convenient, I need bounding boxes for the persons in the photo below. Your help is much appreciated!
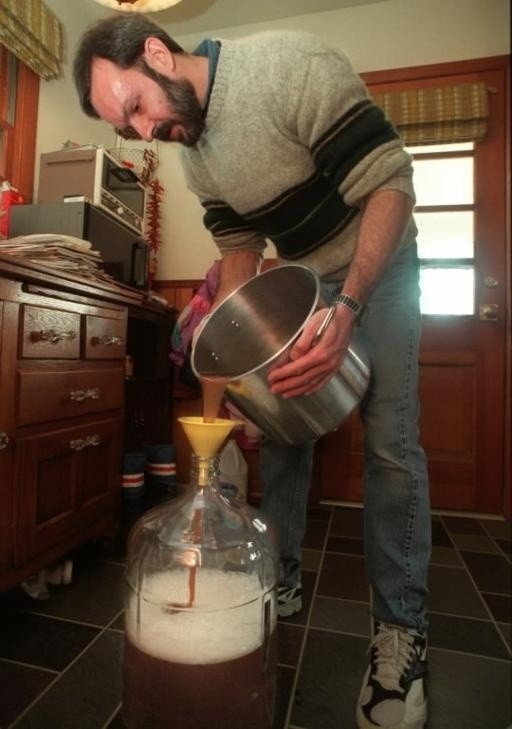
[70,5,437,728]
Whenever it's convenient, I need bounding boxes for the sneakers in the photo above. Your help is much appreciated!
[277,578,304,618]
[356,616,430,729]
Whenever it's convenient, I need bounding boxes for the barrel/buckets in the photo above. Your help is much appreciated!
[122,455,282,729]
[216,439,249,504]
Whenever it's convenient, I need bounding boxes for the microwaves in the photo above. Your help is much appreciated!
[7,142,153,293]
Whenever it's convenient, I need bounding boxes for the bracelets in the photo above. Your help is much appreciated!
[332,292,370,327]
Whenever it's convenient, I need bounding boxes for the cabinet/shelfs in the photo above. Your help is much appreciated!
[1,252,178,599]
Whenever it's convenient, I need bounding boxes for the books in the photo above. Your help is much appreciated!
[0,232,117,286]
